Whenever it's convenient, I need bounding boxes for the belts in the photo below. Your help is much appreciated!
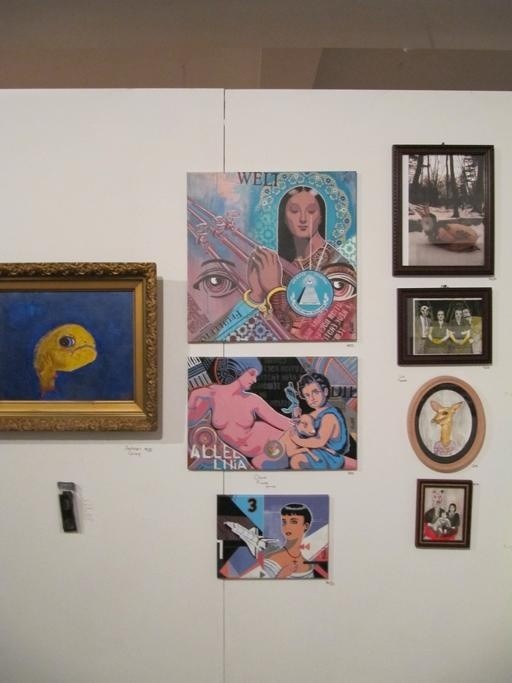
[421,337,429,339]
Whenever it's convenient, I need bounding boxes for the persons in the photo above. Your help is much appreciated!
[260,503,315,579]
[287,371,349,470]
[186,355,357,468]
[415,303,430,353]
[198,245,294,342]
[190,247,357,303]
[243,185,353,340]
[464,307,482,353]
[424,489,448,529]
[447,503,460,534]
[447,307,471,353]
[434,511,454,534]
[422,214,479,251]
[424,309,450,353]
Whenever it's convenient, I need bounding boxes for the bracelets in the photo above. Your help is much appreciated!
[266,286,286,312]
[242,288,268,311]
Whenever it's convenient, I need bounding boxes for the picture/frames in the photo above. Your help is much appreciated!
[0,260,159,434]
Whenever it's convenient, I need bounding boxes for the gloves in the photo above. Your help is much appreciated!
[432,337,442,344]
[450,334,459,344]
[458,336,468,345]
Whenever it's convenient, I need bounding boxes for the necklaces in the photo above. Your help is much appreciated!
[283,545,302,562]
[294,241,325,262]
